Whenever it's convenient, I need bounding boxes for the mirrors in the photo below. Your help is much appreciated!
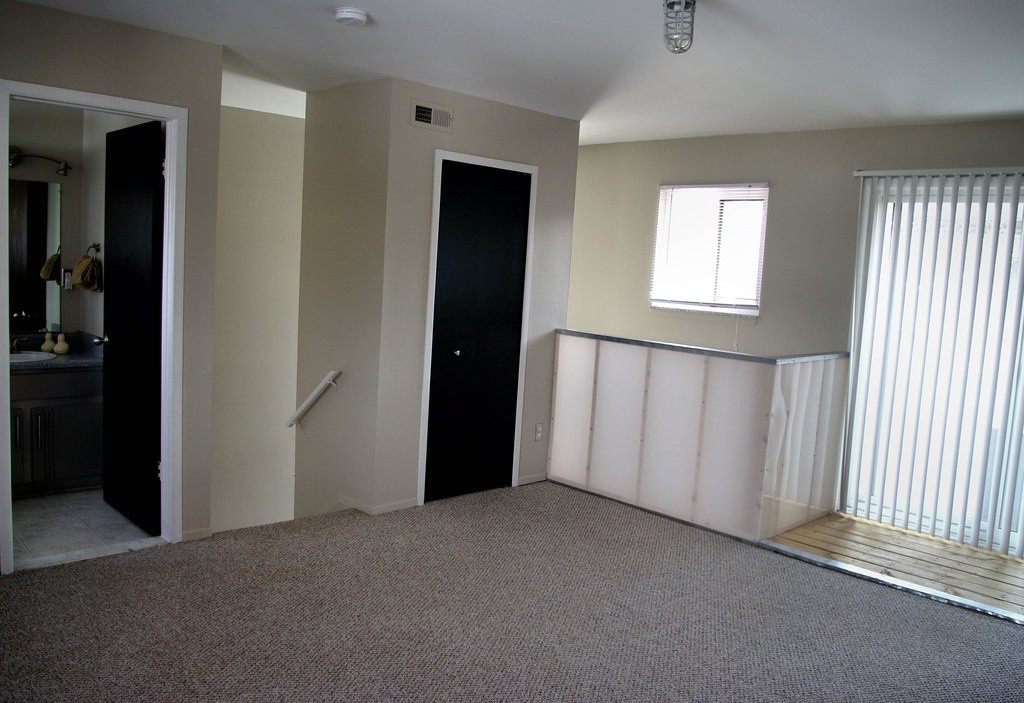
[8,179,63,333]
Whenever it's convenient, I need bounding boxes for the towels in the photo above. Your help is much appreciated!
[70,255,103,294]
[40,253,61,286]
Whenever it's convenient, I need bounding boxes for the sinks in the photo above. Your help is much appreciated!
[10,350,57,362]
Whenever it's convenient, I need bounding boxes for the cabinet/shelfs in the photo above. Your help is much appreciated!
[10,367,104,500]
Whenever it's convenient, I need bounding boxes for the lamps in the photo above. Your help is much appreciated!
[56,160,69,176]
[663,0,697,55]
[8,152,20,167]
